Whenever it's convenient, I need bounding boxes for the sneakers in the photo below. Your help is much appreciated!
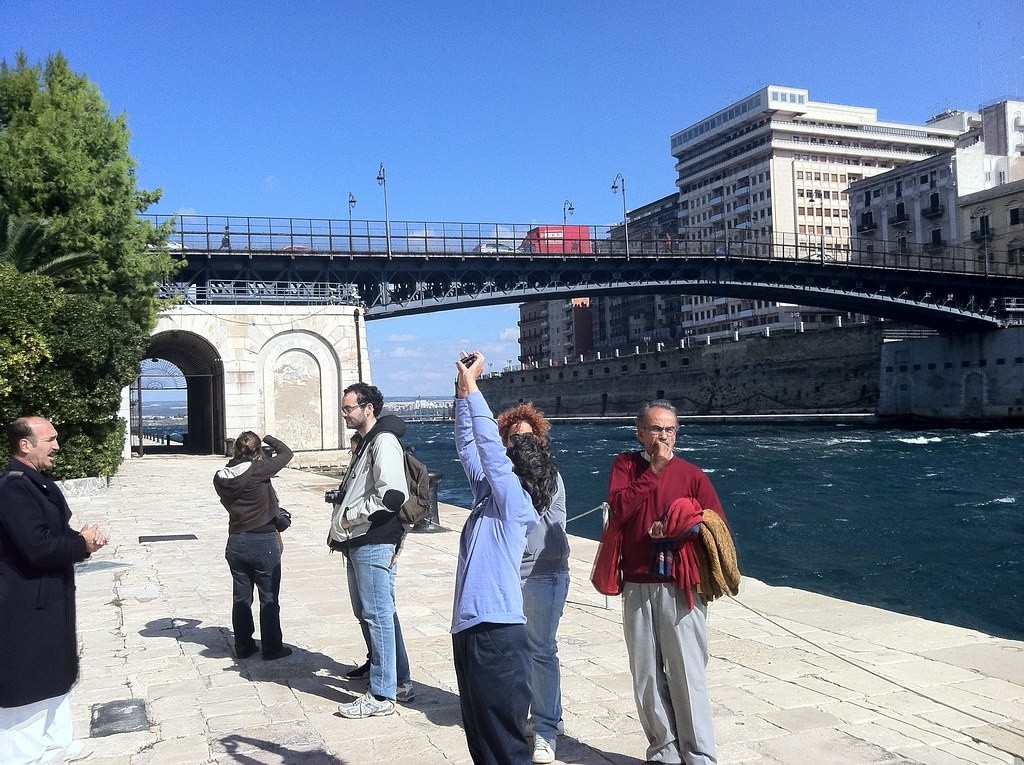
[531,732,557,763]
[338,691,396,719]
[524,718,564,737]
[366,680,416,702]
[262,646,292,661]
[344,660,370,680]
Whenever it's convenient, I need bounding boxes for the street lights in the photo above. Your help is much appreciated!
[562,199,575,257]
[610,173,630,263]
[970,207,989,278]
[348,191,356,260]
[808,187,824,266]
[376,161,392,260]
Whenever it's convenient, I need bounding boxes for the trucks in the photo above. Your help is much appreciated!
[518,224,591,254]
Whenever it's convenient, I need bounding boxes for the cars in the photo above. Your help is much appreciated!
[708,246,741,255]
[146,239,188,251]
[282,244,311,252]
[474,243,522,254]
[805,254,836,262]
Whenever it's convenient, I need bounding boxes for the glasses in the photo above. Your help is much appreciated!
[505,432,533,444]
[642,425,677,437]
[339,402,368,415]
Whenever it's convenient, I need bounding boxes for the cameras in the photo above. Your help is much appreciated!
[459,350,475,363]
[325,490,346,505]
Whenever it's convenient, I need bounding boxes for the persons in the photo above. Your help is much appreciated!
[608,399,729,765]
[450,351,559,765]
[0,417,107,765]
[345,432,372,689]
[326,382,416,718]
[213,431,292,660]
[218,226,231,250]
[498,403,571,763]
[663,233,673,253]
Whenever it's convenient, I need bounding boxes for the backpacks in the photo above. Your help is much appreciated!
[368,431,431,524]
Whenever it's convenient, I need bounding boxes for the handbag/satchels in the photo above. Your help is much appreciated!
[274,507,293,532]
[589,451,633,596]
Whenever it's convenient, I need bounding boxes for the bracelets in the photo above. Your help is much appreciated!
[458,386,467,391]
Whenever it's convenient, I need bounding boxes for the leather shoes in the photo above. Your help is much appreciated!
[237,645,259,659]
